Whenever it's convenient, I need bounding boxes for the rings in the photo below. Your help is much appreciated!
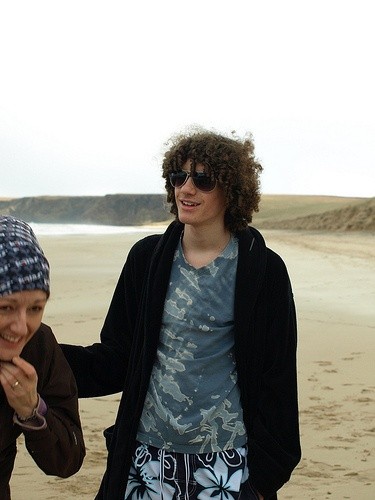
[11,380,19,388]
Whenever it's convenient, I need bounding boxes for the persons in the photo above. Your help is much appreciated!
[0,216,86,500]
[58,133,301,500]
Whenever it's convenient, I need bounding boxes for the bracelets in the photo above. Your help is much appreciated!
[17,406,38,422]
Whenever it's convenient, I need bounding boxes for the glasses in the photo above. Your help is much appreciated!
[169,169,218,193]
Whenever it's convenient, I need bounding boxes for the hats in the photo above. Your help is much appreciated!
[0,215,50,300]
